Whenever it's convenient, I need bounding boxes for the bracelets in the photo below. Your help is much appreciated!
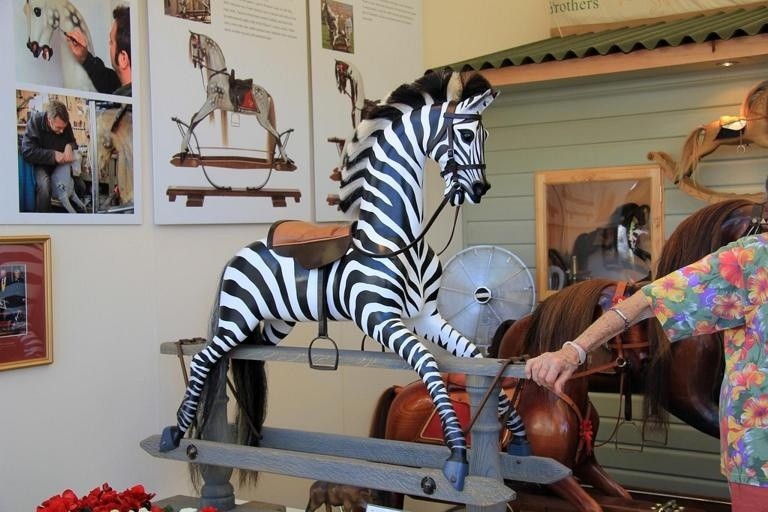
[610,308,630,331]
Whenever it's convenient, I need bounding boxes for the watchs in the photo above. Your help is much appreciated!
[563,341,586,366]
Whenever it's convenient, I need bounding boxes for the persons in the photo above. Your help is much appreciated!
[20,102,86,213]
[0,265,27,333]
[524,233,767,512]
[65,7,132,99]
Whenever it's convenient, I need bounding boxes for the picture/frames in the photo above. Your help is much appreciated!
[0,233,54,374]
[533,162,665,311]
